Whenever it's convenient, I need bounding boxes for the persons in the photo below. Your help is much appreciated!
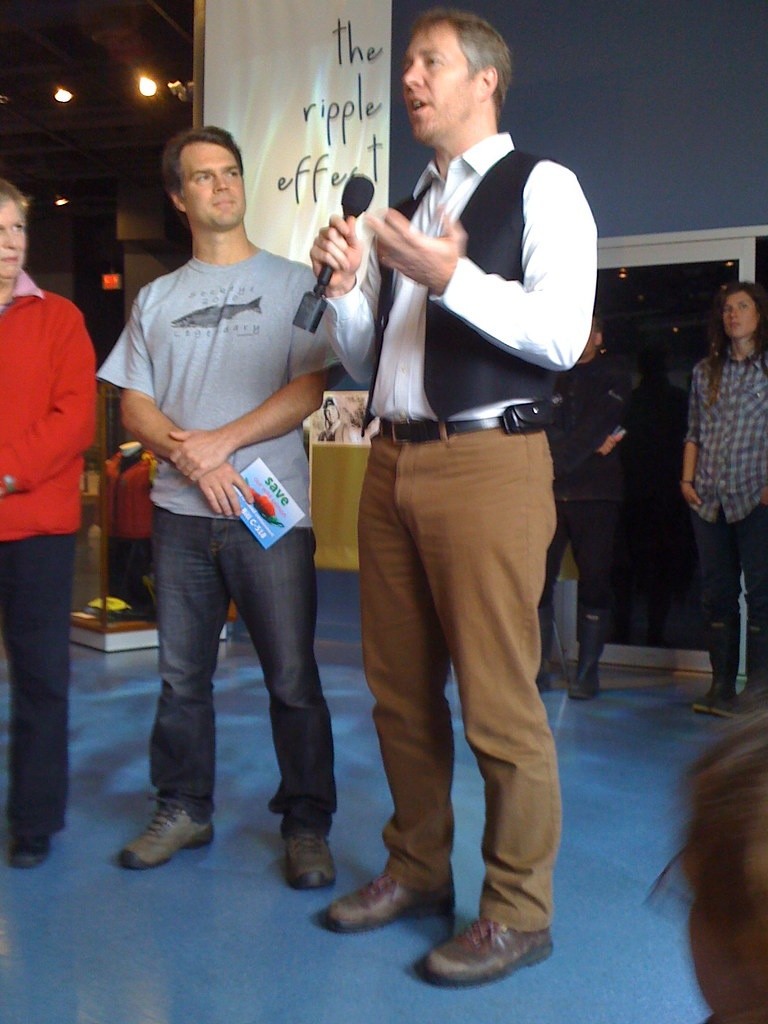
[0,178,97,869]
[311,9,597,988]
[681,283,768,720]
[530,307,631,700]
[95,126,338,889]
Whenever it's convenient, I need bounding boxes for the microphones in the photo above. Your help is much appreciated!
[292,175,375,335]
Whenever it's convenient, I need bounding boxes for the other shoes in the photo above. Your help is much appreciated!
[7,834,49,867]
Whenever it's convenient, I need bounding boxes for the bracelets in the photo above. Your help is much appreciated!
[680,481,689,485]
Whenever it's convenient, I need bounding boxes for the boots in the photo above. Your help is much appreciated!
[711,619,768,717]
[568,608,609,699]
[693,622,740,712]
[536,606,567,691]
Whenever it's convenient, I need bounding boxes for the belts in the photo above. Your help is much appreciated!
[378,417,503,443]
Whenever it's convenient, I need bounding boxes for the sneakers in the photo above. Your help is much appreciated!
[120,795,213,870]
[285,829,335,888]
[328,872,456,932]
[424,919,552,988]
